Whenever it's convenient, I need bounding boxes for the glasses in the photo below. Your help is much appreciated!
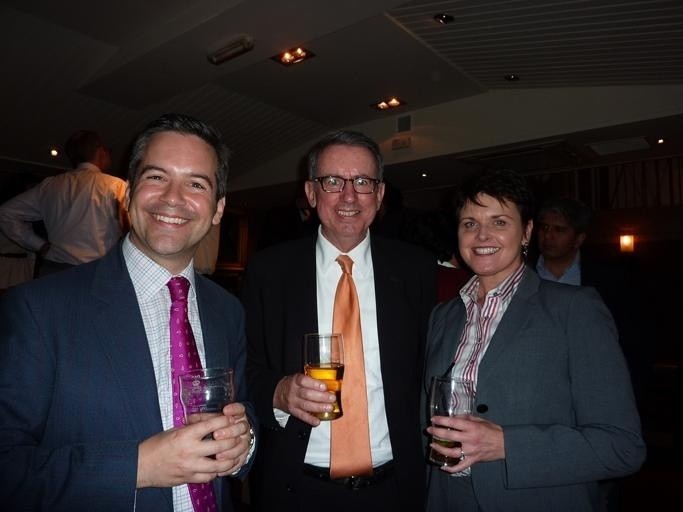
[309,176,384,194]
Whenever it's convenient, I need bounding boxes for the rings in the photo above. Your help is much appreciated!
[459,445,465,461]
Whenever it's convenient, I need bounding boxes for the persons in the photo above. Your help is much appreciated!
[240,129,439,512]
[2,132,133,279]
[414,165,647,512]
[0,111,260,512]
[526,189,607,292]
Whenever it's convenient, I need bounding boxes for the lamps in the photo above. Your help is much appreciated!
[208,35,256,65]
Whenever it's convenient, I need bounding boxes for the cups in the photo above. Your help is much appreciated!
[304,333,345,422]
[179,370,240,460]
[427,373,472,467]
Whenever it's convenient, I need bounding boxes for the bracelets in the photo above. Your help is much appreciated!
[249,427,255,447]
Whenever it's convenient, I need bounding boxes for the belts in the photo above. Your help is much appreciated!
[302,460,398,488]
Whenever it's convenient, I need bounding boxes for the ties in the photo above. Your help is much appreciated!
[330,255,373,481]
[166,277,218,512]
